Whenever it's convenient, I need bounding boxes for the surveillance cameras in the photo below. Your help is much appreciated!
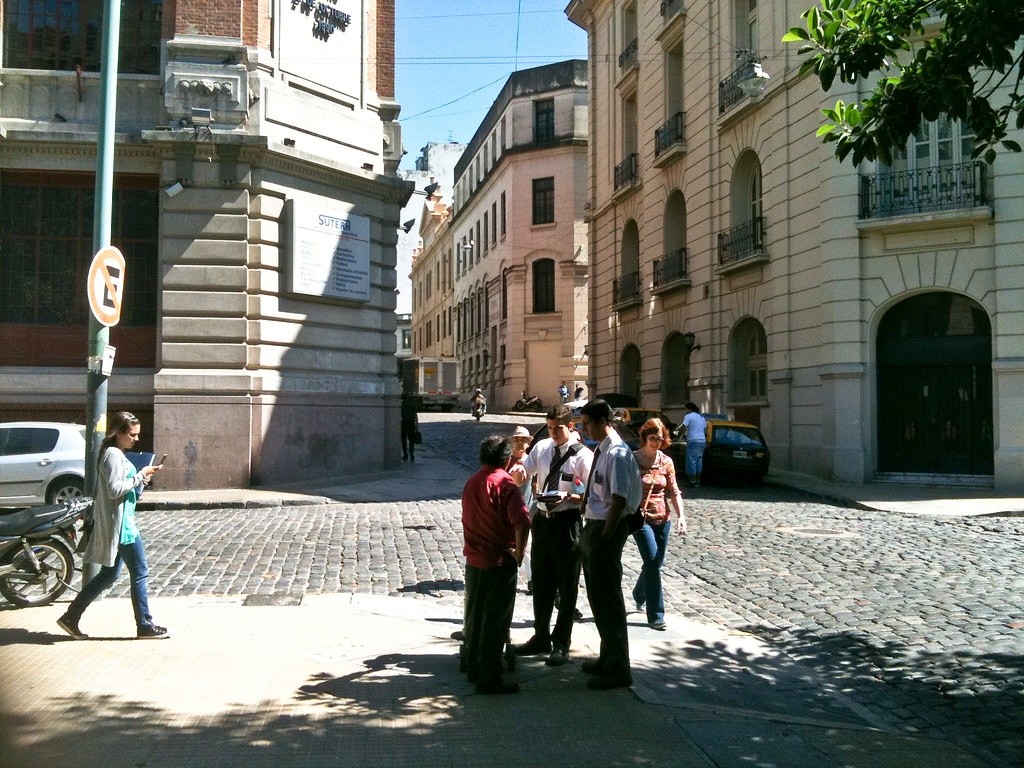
[166,183,184,197]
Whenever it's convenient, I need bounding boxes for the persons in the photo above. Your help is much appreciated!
[508,406,594,666]
[462,436,532,694]
[558,381,570,405]
[57,412,173,641]
[451,426,533,642]
[672,402,707,488]
[632,418,689,630]
[400,391,419,461]
[471,387,486,417]
[575,383,584,401]
[579,399,643,691]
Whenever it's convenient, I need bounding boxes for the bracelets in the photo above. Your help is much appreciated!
[511,548,516,556]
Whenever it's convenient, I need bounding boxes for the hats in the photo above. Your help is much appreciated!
[512,426,533,441]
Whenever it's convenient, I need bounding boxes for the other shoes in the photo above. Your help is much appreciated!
[648,623,666,629]
[589,673,633,689]
[480,680,519,694]
[581,658,602,674]
[468,671,504,682]
[637,603,644,612]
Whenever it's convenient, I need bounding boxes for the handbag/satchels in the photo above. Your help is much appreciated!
[414,429,422,444]
[625,508,645,534]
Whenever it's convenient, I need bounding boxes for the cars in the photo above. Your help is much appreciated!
[522,410,649,458]
[611,406,677,436]
[563,396,589,410]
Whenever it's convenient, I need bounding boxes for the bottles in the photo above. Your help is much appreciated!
[574,479,586,495]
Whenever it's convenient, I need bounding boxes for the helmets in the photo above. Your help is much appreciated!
[476,389,481,393]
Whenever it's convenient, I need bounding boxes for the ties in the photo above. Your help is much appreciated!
[581,447,600,513]
[545,446,561,512]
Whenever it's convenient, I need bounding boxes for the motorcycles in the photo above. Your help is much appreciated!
[0,495,94,607]
[516,390,543,412]
[468,395,487,421]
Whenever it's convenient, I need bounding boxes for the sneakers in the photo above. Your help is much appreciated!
[137,626,168,638]
[57,615,89,639]
[514,635,551,655]
[545,648,569,666]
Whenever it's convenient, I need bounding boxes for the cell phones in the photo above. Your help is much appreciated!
[157,453,168,466]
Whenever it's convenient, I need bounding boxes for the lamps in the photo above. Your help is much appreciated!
[461,242,472,252]
[413,181,438,201]
[398,218,415,234]
[736,49,770,97]
[684,331,701,356]
[191,107,215,134]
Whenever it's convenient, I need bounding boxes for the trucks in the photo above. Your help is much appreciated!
[402,355,461,413]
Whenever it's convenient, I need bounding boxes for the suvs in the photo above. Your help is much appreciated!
[668,412,771,490]
[0,420,88,510]
